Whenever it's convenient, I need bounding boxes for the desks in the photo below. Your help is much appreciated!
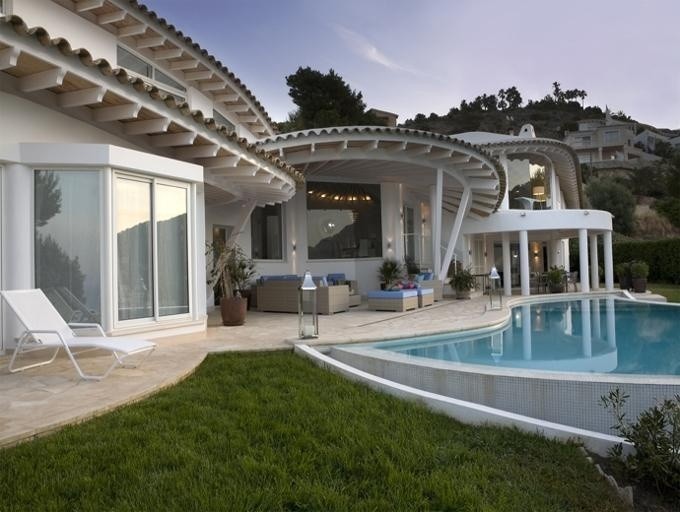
[367,288,434,311]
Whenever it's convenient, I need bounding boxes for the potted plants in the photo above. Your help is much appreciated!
[547,265,564,292]
[205,241,256,326]
[615,260,650,293]
[376,255,426,291]
[447,264,481,299]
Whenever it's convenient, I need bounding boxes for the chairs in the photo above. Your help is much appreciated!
[536,271,578,294]
[1,288,157,381]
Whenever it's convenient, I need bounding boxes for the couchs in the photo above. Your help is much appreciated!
[251,273,361,314]
